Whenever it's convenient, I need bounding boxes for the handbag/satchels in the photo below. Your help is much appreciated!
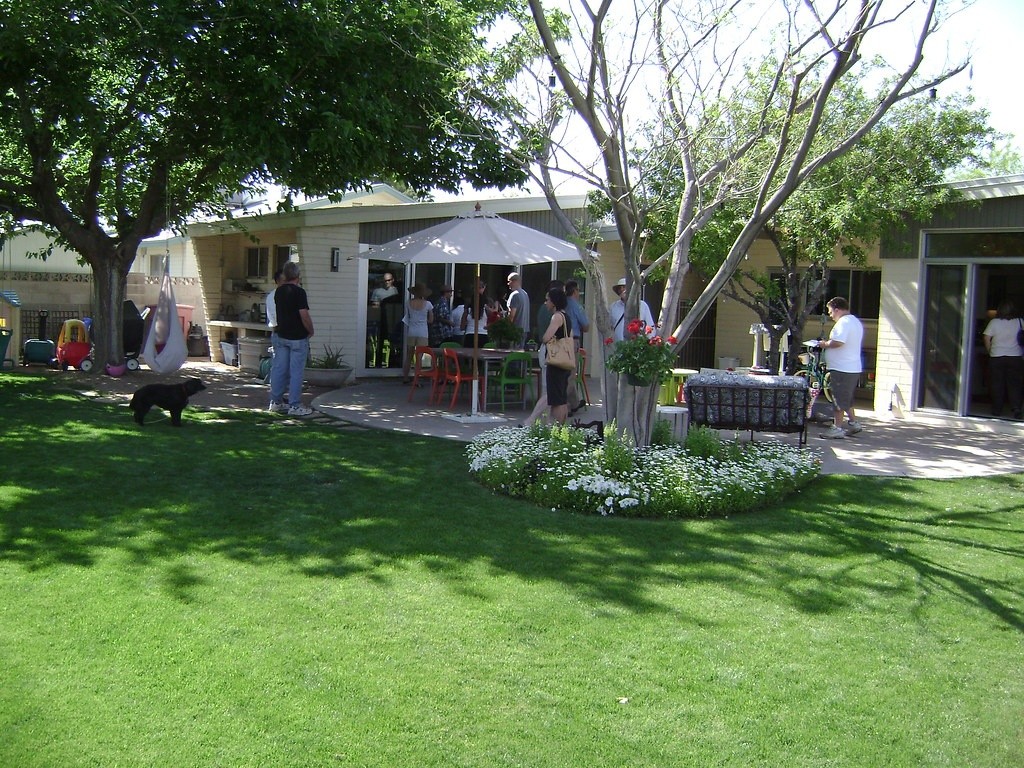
[544,311,576,370]
[1018,319,1024,346]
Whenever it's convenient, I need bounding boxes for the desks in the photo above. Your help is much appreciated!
[657,368,699,404]
[427,347,542,411]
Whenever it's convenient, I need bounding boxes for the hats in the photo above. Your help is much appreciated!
[439,285,455,292]
[408,282,432,297]
[613,278,626,295]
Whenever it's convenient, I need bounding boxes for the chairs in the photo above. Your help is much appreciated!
[408,341,593,413]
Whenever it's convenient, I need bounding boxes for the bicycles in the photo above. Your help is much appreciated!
[793,346,833,403]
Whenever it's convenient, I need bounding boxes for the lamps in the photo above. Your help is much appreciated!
[330,246,340,271]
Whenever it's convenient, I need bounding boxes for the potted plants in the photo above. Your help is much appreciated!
[484,316,525,353]
[303,345,352,386]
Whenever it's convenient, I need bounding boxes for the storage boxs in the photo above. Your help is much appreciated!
[237,338,273,372]
[220,342,238,366]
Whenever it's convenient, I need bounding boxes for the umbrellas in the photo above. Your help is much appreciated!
[346,202,599,414]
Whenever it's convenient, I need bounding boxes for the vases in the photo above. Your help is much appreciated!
[627,371,648,386]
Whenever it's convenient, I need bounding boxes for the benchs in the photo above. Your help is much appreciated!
[681,377,810,450]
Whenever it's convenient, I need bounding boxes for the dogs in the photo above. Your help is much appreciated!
[128,378,211,428]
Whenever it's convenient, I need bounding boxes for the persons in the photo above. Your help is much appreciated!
[818,297,863,439]
[453,279,507,347]
[434,285,456,346]
[266,262,313,415]
[402,283,433,387]
[518,281,589,429]
[369,273,398,368]
[507,272,529,348]
[608,278,657,340]
[983,302,1024,418]
[542,288,572,425]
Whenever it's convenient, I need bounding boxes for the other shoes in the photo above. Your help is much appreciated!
[404,379,411,383]
[992,409,1000,415]
[1014,408,1021,418]
[571,400,585,412]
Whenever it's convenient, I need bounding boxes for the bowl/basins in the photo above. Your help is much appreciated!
[106,364,126,376]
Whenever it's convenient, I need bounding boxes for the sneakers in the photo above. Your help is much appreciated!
[845,421,862,435]
[269,400,289,411]
[288,405,312,415]
[820,424,844,439]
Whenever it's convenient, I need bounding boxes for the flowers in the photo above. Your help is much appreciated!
[604,322,683,385]
